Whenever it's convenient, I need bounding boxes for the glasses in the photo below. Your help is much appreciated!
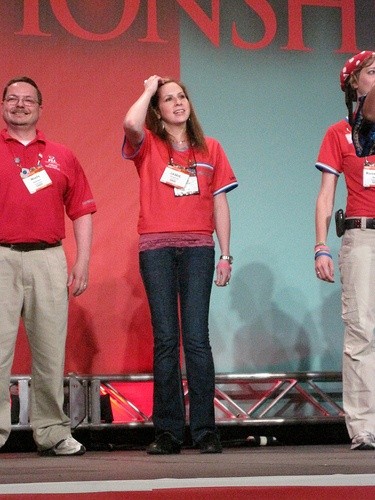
[4,96,40,106]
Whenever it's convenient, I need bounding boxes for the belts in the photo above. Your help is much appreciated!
[344,218,375,229]
[0,241,62,252]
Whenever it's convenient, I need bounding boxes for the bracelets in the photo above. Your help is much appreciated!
[314,243,332,260]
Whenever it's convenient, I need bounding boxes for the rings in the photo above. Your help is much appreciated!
[226,282,228,284]
[317,271,320,274]
[84,285,87,286]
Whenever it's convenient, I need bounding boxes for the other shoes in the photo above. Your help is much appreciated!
[147,437,181,454]
[199,435,222,453]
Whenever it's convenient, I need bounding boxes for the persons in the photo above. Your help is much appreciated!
[313,50,375,449]
[352,83,375,157]
[120,74,238,454]
[1,77,98,455]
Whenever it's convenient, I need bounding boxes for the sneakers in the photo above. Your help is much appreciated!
[350,432,375,450]
[37,434,86,456]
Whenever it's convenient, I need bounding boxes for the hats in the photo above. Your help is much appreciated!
[340,51,375,91]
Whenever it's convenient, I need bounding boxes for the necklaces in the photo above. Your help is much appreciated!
[177,140,187,144]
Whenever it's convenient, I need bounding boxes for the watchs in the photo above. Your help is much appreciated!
[219,255,233,264]
